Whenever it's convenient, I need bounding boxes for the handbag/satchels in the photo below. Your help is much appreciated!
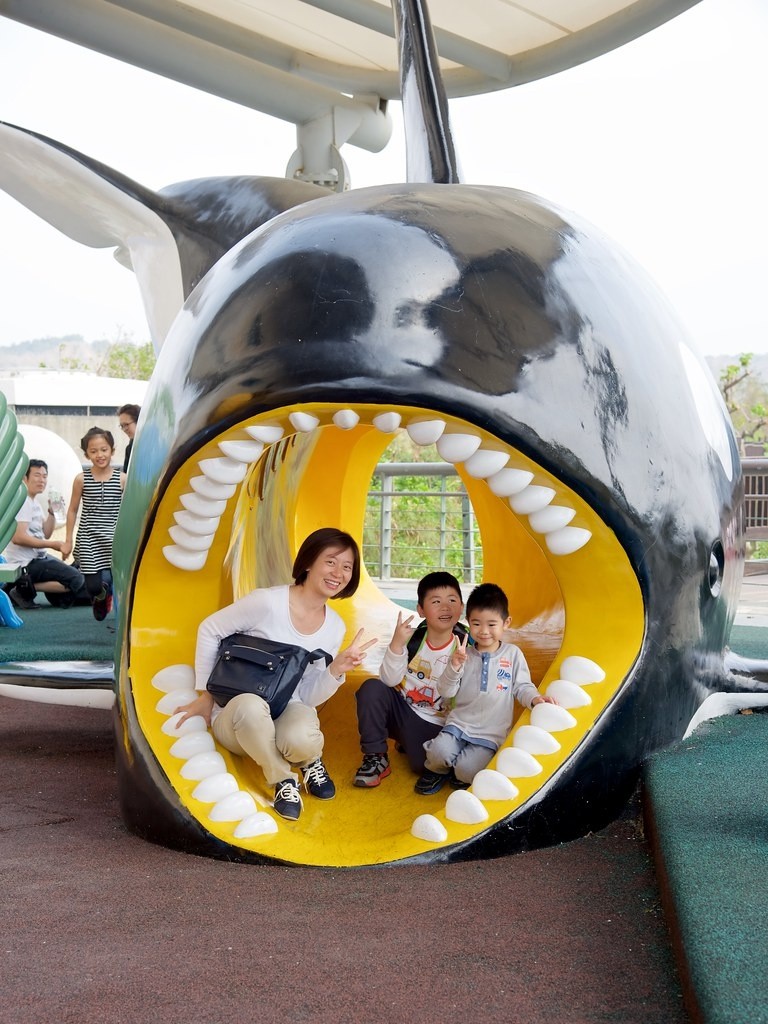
[205,632,315,721]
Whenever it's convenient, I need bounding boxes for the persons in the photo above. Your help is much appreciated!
[116,403,141,474]
[0,456,84,610]
[60,425,128,621]
[353,570,476,788]
[173,528,378,822]
[413,583,560,795]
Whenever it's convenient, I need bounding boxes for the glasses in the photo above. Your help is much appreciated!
[118,420,135,429]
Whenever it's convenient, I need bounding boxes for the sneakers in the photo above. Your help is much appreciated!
[299,757,336,801]
[414,769,453,794]
[394,741,406,753]
[450,775,471,790]
[91,587,108,621]
[9,587,42,611]
[353,752,392,787]
[273,779,305,821]
[104,594,113,613]
